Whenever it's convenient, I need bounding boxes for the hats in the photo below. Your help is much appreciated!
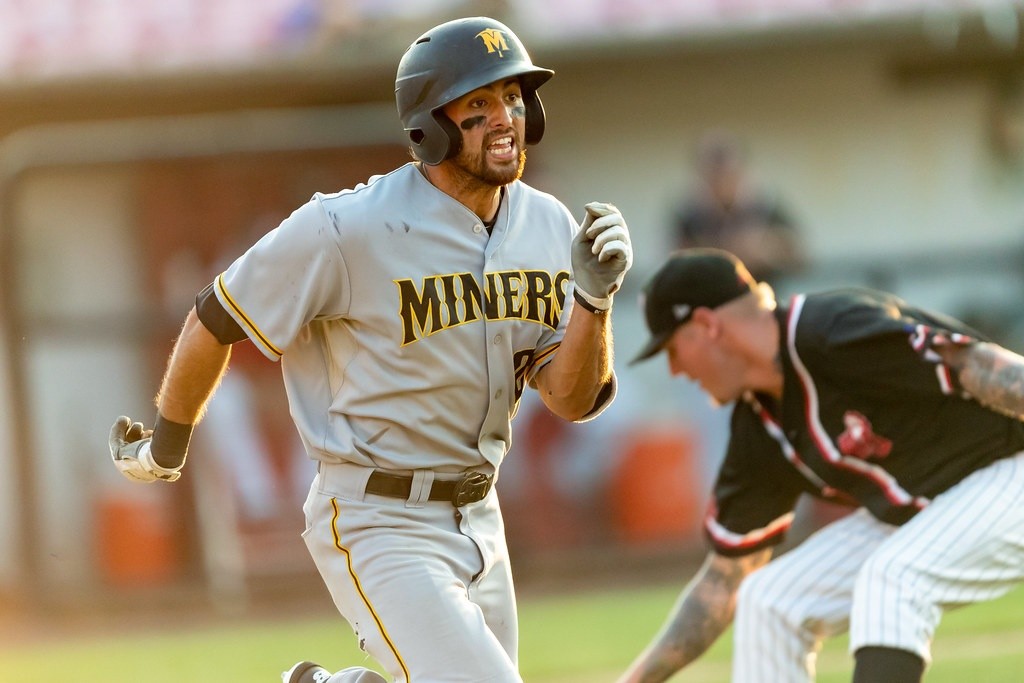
[628,251,756,365]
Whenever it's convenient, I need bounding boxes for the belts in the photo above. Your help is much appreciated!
[316,460,495,507]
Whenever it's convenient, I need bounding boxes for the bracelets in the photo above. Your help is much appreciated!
[573,287,613,316]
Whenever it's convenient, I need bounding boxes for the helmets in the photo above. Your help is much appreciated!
[392,15,556,166]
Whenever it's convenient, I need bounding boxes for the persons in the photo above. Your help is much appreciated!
[616,142,1024,683]
[109,17,634,683]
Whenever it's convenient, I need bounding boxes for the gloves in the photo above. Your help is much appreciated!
[107,412,194,483]
[571,197,634,311]
